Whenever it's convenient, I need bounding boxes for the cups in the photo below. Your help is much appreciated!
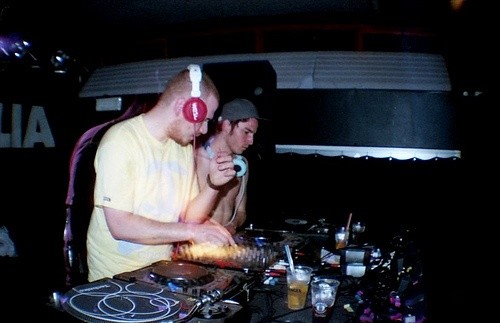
[286,266,312,310]
[336,225,349,251]
[311,277,340,323]
[350,221,364,242]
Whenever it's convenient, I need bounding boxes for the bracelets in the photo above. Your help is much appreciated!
[206,174,224,190]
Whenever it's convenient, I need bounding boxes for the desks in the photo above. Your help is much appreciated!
[234,241,427,323]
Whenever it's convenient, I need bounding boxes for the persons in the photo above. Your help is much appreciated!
[192,100,270,236]
[85,68,239,282]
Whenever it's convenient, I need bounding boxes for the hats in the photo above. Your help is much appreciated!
[220,98,271,122]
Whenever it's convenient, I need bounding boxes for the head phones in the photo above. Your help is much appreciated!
[182,64,208,124]
[204,136,248,177]
